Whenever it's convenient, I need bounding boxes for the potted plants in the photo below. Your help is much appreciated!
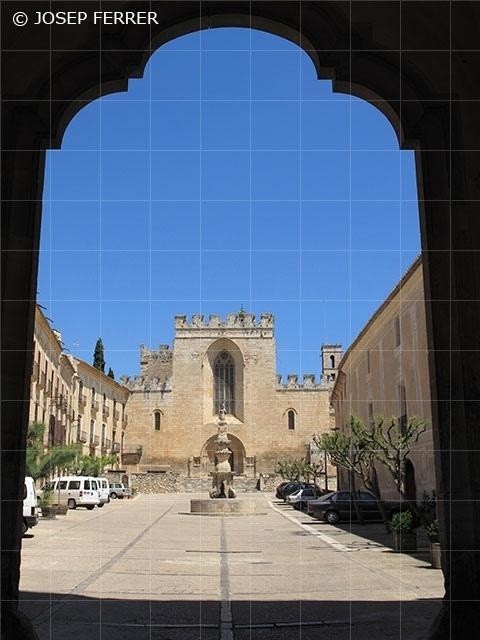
[38,487,56,519]
[388,510,417,551]
[424,519,442,568]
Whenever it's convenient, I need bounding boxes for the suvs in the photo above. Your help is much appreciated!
[109,482,130,499]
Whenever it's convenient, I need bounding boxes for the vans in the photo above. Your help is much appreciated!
[22,477,40,538]
[37,476,101,510]
[95,477,111,507]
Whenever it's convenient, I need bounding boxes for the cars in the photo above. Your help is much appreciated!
[275,481,335,511]
[307,490,410,525]
